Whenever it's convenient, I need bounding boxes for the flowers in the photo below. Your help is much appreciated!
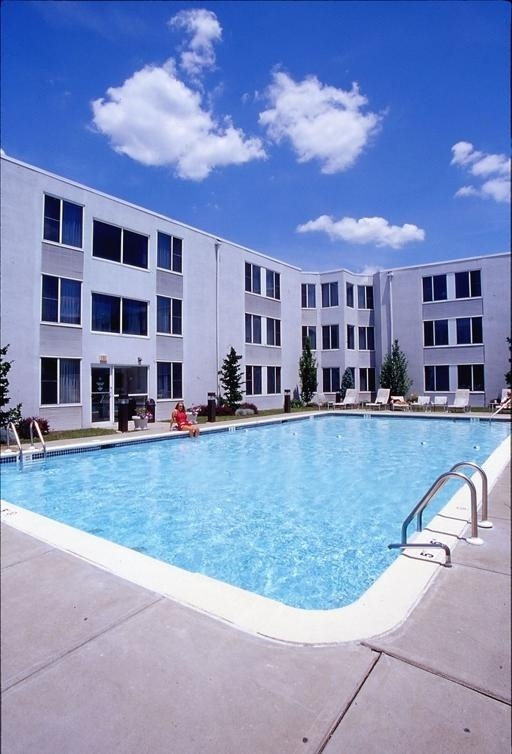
[134,407,154,420]
[188,403,204,424]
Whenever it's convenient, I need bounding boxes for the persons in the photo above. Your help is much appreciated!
[169,402,199,439]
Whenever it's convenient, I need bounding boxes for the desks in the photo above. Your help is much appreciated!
[489,403,499,415]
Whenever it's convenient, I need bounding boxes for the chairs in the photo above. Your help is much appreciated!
[365,388,391,411]
[448,389,471,414]
[496,388,511,413]
[389,396,449,412]
[333,389,359,410]
[316,390,333,411]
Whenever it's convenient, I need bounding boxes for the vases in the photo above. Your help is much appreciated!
[185,412,199,424]
[132,416,148,429]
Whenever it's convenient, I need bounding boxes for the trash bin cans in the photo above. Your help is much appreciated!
[146,399,155,423]
